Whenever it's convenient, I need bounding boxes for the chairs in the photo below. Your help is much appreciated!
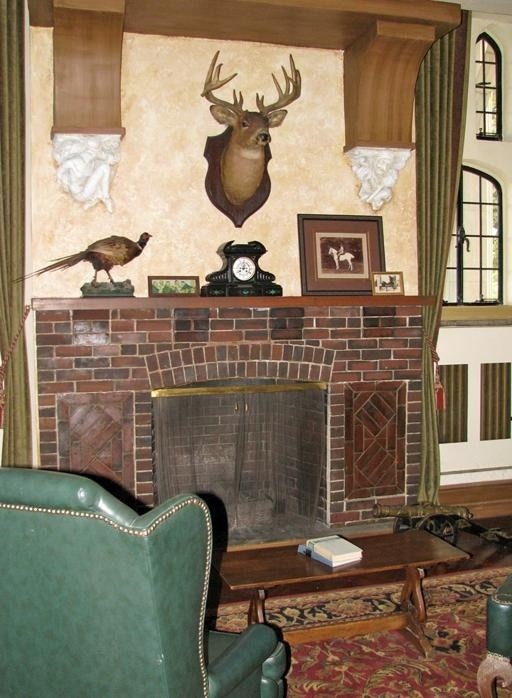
[0,468,288,698]
[476,572,512,698]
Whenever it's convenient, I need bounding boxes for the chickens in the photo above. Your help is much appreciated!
[11,232,153,287]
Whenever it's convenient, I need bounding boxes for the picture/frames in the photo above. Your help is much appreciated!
[298,214,386,296]
[371,271,404,297]
[148,276,200,298]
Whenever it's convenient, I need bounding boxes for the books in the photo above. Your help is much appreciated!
[297,543,362,568]
[303,533,363,562]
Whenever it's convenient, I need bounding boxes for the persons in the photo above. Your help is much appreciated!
[336,242,345,260]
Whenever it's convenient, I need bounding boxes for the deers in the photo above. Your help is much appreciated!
[201,50,301,207]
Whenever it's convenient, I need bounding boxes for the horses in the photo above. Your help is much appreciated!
[328,247,355,272]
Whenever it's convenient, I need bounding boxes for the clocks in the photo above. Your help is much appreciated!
[201,240,283,296]
